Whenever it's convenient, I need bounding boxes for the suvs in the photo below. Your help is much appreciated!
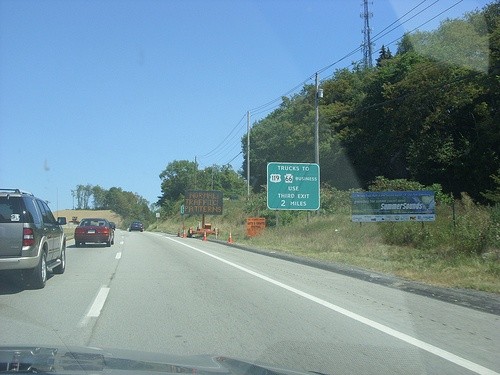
[0,189,67,289]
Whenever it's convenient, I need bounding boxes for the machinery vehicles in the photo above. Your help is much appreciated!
[188,224,219,238]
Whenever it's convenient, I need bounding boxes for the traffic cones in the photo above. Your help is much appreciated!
[182,230,187,238]
[202,232,207,241]
[227,232,233,243]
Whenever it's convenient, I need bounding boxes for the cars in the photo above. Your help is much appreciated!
[110,222,115,230]
[129,221,143,232]
[74,218,114,247]
